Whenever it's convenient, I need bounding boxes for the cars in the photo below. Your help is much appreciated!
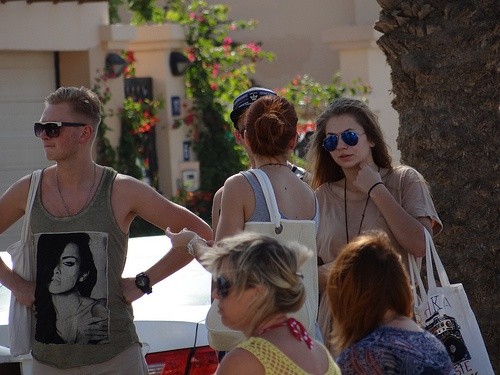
[0,235,224,375]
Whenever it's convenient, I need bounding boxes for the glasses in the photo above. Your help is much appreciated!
[322,131,366,152]
[34,121,87,137]
[215,275,231,298]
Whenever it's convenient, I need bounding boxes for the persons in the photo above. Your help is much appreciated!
[199,232,343,375]
[0,87,214,375]
[206,87,322,356]
[166,95,320,358]
[325,230,457,375]
[307,97,443,362]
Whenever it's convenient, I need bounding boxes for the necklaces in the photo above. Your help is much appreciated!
[257,163,287,168]
[343,175,369,245]
[56,163,97,216]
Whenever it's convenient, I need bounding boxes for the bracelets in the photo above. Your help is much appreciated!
[367,182,384,196]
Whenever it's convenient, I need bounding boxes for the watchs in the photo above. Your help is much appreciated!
[135,272,152,295]
[186,235,207,258]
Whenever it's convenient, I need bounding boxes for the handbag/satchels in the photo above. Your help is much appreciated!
[206,168,318,350]
[6,169,43,357]
[407,227,495,375]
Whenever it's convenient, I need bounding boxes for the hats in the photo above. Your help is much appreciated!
[229,86,277,126]
